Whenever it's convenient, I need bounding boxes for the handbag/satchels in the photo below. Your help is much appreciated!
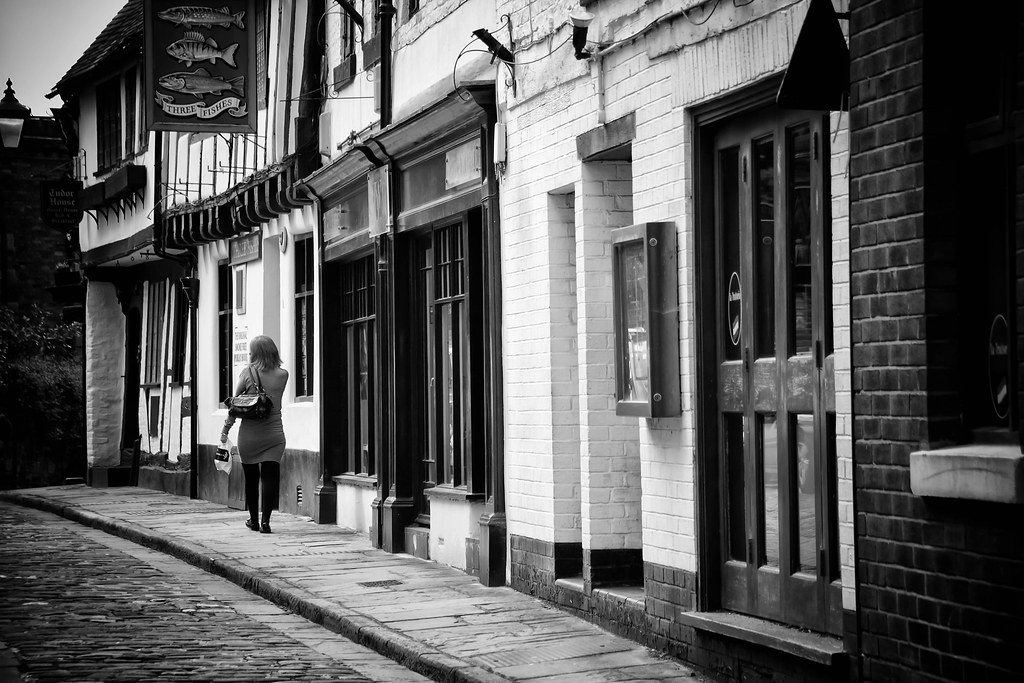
[224,366,274,419]
[213,439,233,476]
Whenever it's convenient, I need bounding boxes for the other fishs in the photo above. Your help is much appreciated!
[158,3,246,101]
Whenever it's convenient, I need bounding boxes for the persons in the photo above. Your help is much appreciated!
[220,336,288,532]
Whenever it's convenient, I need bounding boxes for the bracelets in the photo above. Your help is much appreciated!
[221,433,228,436]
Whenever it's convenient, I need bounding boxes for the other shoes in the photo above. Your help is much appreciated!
[260,522,271,533]
[245,519,259,531]
